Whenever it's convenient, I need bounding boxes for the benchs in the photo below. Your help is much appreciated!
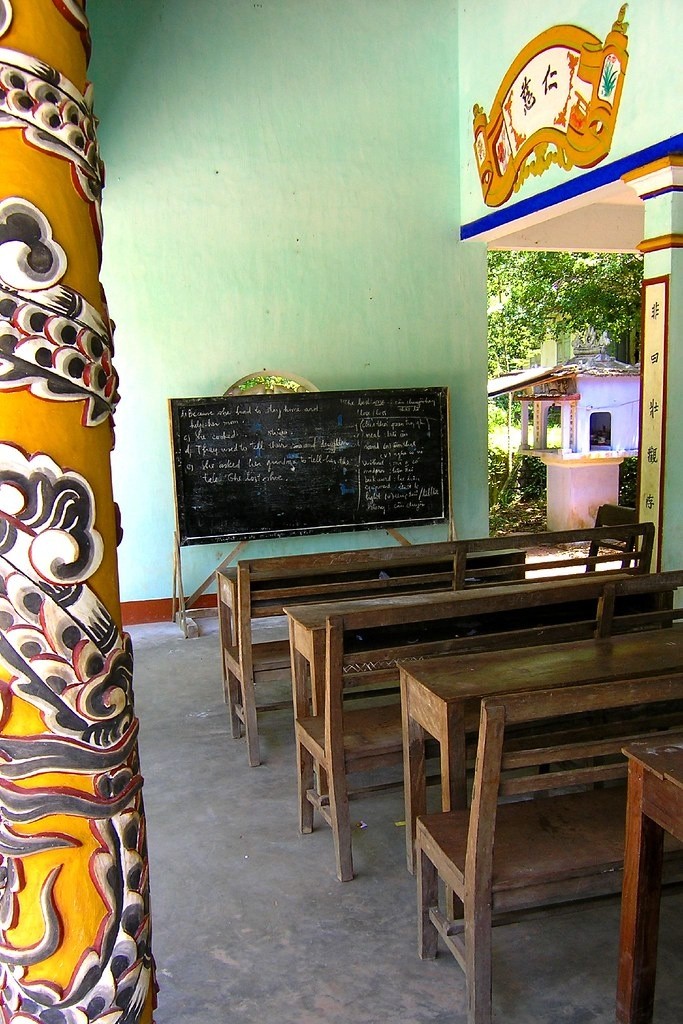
[223,523,655,768]
[409,673,683,1024]
[296,568,683,882]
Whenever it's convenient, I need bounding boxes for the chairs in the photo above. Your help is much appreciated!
[586,505,636,572]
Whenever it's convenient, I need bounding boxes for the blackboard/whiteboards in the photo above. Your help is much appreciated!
[167,386,453,546]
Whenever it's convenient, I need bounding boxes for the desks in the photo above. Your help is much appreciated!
[283,574,635,796]
[215,547,529,681]
[602,736,683,1024]
[395,627,683,922]
